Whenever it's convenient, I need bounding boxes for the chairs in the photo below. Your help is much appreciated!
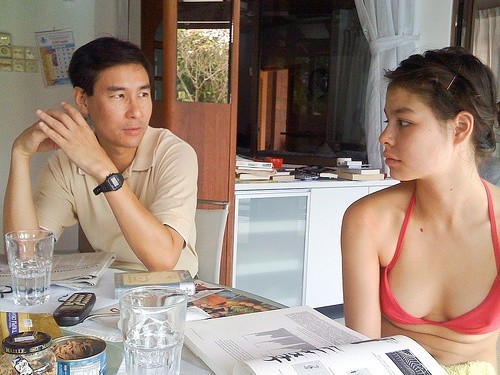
[195,198,231,284]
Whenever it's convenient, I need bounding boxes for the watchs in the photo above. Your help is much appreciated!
[93,173,124,195]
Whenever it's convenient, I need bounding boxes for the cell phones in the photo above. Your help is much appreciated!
[52,292,96,327]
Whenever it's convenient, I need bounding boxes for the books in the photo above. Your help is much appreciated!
[183,305,445,375]
[236,156,294,181]
[329,166,384,181]
[114,270,196,299]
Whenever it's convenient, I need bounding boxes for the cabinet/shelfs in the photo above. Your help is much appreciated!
[232,181,400,309]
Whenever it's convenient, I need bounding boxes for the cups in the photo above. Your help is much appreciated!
[119,285,189,375]
[52,334,107,375]
[4,229,55,307]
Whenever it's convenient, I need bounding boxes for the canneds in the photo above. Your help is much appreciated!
[0,331,56,375]
[52,334,108,375]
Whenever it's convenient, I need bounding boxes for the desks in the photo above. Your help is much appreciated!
[0,253,294,375]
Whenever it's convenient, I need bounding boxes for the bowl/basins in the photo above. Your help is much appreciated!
[266,157,283,170]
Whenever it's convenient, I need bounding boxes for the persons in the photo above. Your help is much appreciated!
[340,46,500,371]
[2,37,198,280]
